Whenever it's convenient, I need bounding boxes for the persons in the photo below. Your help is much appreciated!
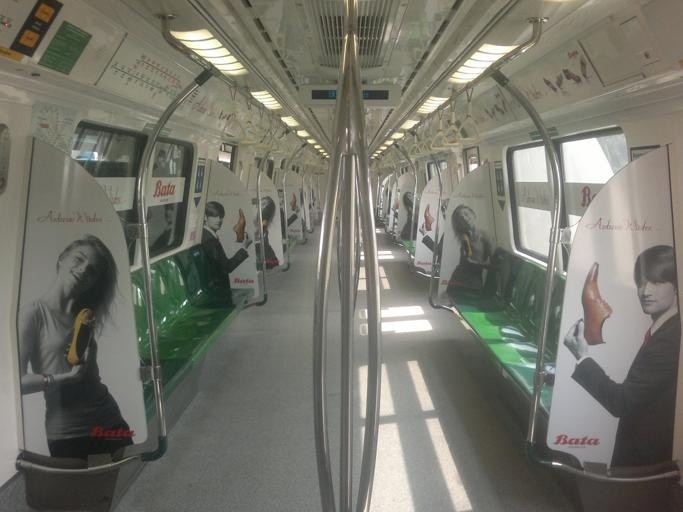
[419,199,449,273]
[153,205,174,250]
[252,197,278,269]
[401,191,417,241]
[446,206,496,297]
[277,189,300,252]
[17,236,138,456]
[562,245,679,466]
[202,202,252,288]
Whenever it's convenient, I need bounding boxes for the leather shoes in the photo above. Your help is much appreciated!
[290,193,296,209]
[582,263,613,345]
[425,204,434,231]
[67,308,96,365]
[233,209,246,242]
[461,233,473,257]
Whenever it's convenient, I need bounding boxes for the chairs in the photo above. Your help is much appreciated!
[445,247,567,422]
[131,243,254,423]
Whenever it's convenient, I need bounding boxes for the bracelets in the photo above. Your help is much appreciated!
[42,374,56,393]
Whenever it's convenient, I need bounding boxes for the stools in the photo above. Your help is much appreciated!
[399,238,417,256]
[282,235,299,254]
